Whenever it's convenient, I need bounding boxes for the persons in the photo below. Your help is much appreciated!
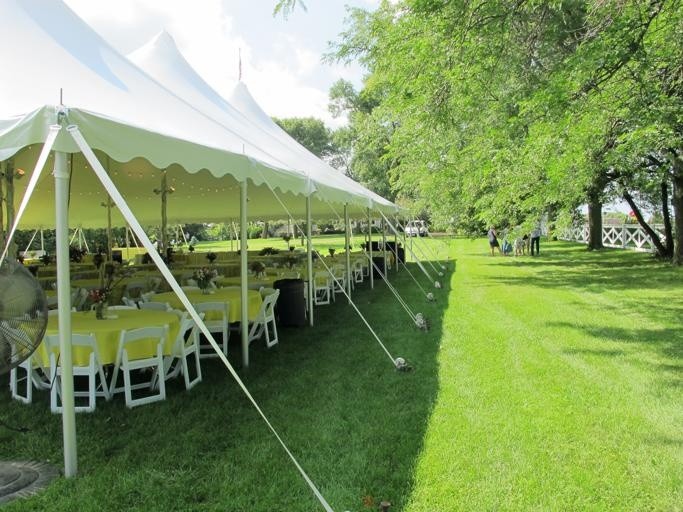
[486,219,540,257]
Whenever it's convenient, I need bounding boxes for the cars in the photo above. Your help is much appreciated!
[403,219,430,239]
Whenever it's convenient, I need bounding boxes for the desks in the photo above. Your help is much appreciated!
[15,311,182,372]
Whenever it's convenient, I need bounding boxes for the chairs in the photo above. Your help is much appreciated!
[107,324,170,410]
[42,332,110,413]
[191,301,230,359]
[5,241,397,321]
[246,289,282,348]
[154,312,207,391]
[2,322,54,406]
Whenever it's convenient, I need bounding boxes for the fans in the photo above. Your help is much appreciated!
[0,255,47,495]
